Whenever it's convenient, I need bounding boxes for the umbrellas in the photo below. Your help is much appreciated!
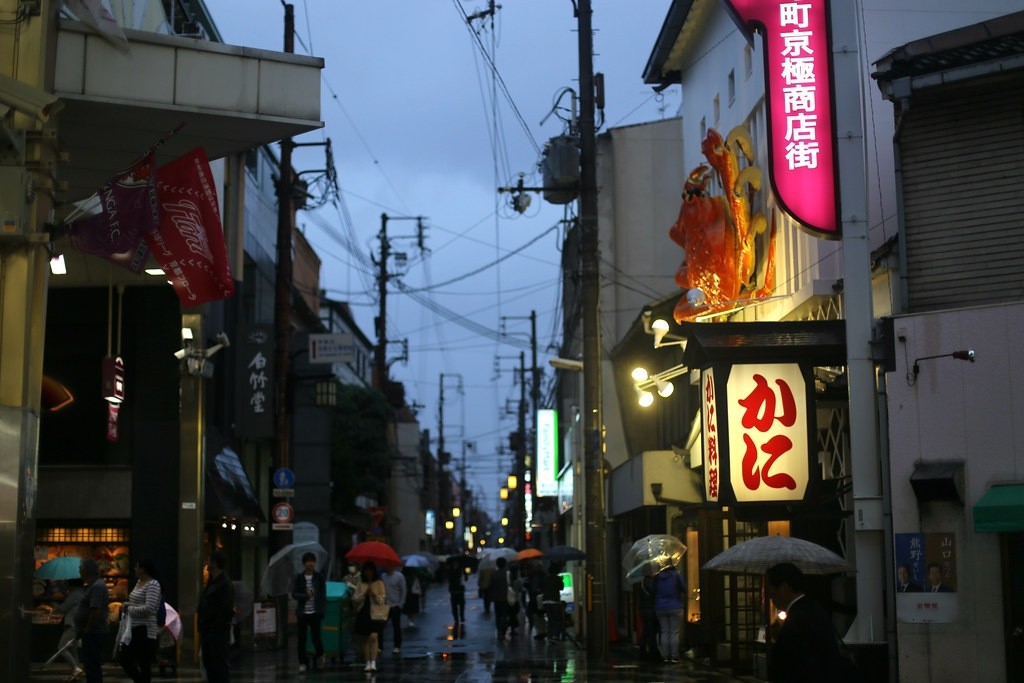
[41,636,75,672]
[229,577,255,624]
[163,601,184,647]
[35,552,86,582]
[259,540,330,598]
[701,533,864,625]
[111,610,127,661]
[621,532,689,577]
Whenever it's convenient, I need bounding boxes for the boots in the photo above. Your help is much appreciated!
[639,637,653,661]
[364,660,370,671]
[648,640,662,661]
[370,660,377,671]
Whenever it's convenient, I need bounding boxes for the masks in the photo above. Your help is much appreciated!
[348,566,356,573]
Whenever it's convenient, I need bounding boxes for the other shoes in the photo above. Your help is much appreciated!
[68,667,86,682]
[316,652,326,669]
[408,618,415,627]
[298,664,307,673]
[392,648,401,653]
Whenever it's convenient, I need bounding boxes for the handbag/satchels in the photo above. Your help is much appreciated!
[144,579,166,627]
[120,611,133,646]
[370,597,391,621]
[506,570,517,606]
[112,612,127,667]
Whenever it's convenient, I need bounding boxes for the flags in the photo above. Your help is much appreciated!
[68,159,161,275]
[143,145,237,313]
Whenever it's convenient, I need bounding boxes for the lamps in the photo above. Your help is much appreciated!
[651,318,687,348]
[912,349,976,378]
[629,363,688,407]
[687,287,794,308]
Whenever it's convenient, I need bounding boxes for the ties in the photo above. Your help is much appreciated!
[901,585,905,592]
[933,586,936,591]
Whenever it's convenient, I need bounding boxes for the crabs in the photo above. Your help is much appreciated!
[670,128,779,324]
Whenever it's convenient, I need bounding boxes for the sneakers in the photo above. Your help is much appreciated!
[664,656,671,662]
[671,657,679,663]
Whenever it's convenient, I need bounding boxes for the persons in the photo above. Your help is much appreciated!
[761,560,847,683]
[195,551,234,683]
[120,556,166,683]
[922,562,954,593]
[290,552,328,673]
[157,620,180,679]
[655,565,689,664]
[342,539,583,641]
[896,564,923,594]
[635,576,666,665]
[349,557,391,674]
[52,579,90,682]
[74,558,111,683]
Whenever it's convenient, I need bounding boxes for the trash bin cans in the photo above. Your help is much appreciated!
[304,581,356,666]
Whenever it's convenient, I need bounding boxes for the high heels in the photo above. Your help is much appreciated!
[534,633,547,640]
